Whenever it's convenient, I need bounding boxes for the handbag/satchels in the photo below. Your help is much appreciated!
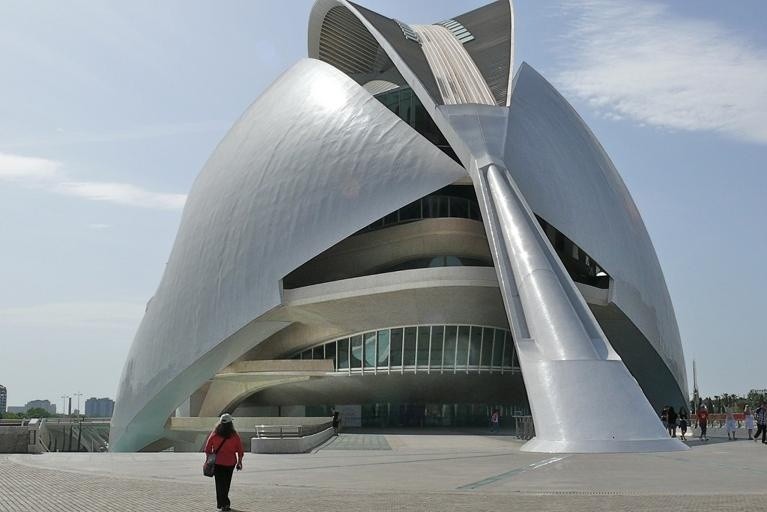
[203,454,215,477]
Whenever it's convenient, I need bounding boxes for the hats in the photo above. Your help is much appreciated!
[220,414,235,423]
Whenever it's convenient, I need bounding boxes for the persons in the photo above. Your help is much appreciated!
[331,408,336,417]
[204,413,243,511]
[743,403,755,440]
[677,406,689,441]
[667,406,678,437]
[696,403,710,442]
[491,409,499,433]
[725,402,738,440]
[333,411,341,436]
[661,405,670,430]
[752,401,767,444]
